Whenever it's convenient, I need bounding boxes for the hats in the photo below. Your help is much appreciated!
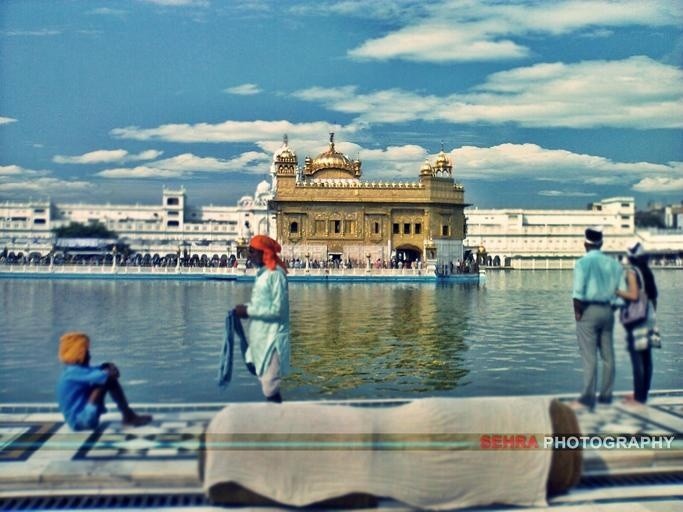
[583,228,602,247]
[627,242,646,258]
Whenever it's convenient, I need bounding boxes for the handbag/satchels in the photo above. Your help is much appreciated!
[619,267,649,326]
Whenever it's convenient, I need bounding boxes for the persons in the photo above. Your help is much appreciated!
[56,330,153,432]
[228,234,291,401]
[1,247,478,276]
[612,240,659,409]
[572,227,624,404]
